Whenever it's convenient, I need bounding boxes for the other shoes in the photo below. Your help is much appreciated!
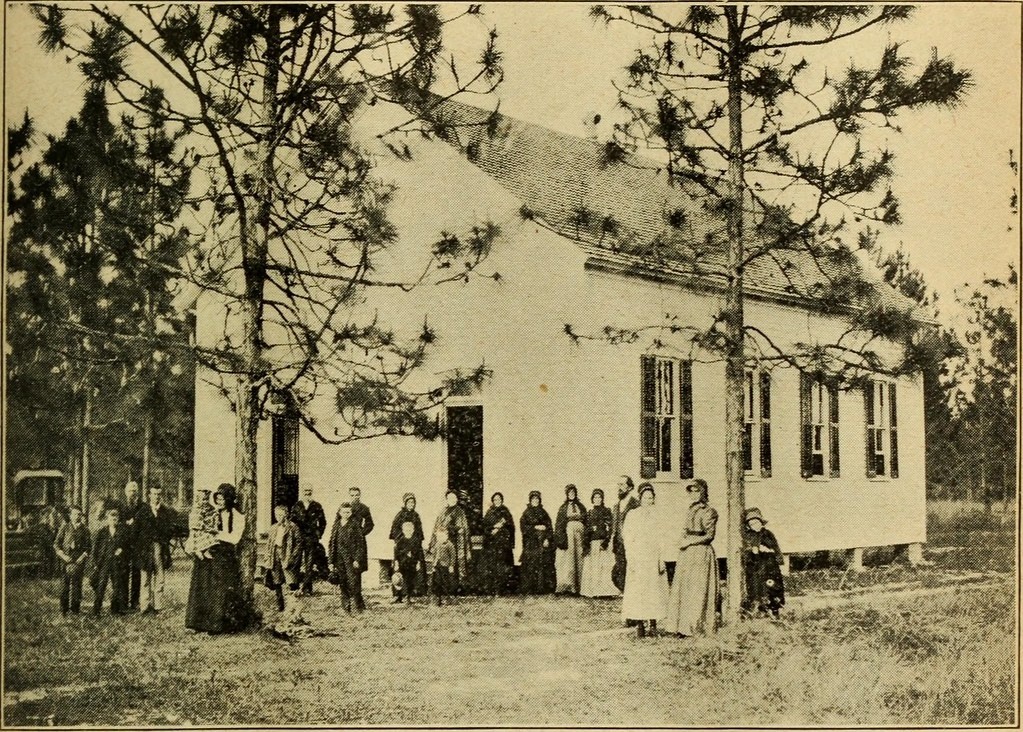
[638,626,646,638]
[63,603,167,618]
[648,625,658,636]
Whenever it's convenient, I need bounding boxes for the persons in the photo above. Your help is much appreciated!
[741,508,785,620]
[621,480,672,639]
[16,475,640,637]
[663,478,721,640]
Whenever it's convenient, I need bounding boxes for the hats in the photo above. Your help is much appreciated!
[638,482,655,498]
[590,490,605,505]
[745,508,769,525]
[64,559,85,578]
[391,572,404,591]
[213,483,236,533]
[90,569,100,590]
[686,478,709,503]
